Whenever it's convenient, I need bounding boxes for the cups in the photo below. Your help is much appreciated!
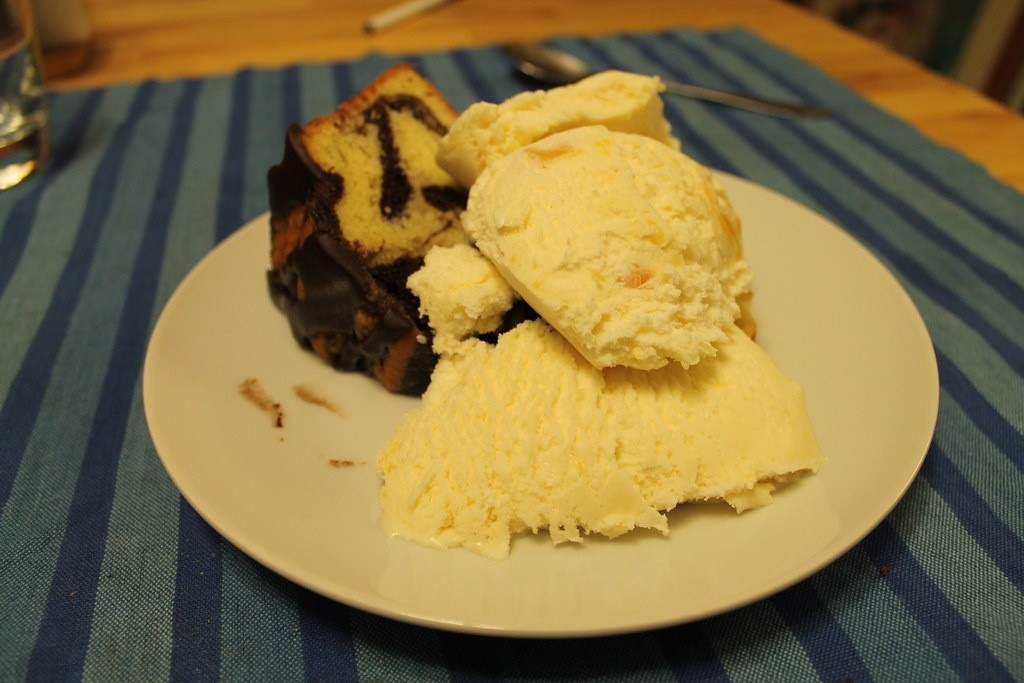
[0,0,50,192]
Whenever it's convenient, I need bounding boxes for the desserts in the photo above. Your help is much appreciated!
[263,65,821,556]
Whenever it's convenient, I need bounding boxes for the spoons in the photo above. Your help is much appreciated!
[500,40,835,124]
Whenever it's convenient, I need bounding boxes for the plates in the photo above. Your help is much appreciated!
[142,169,940,639]
